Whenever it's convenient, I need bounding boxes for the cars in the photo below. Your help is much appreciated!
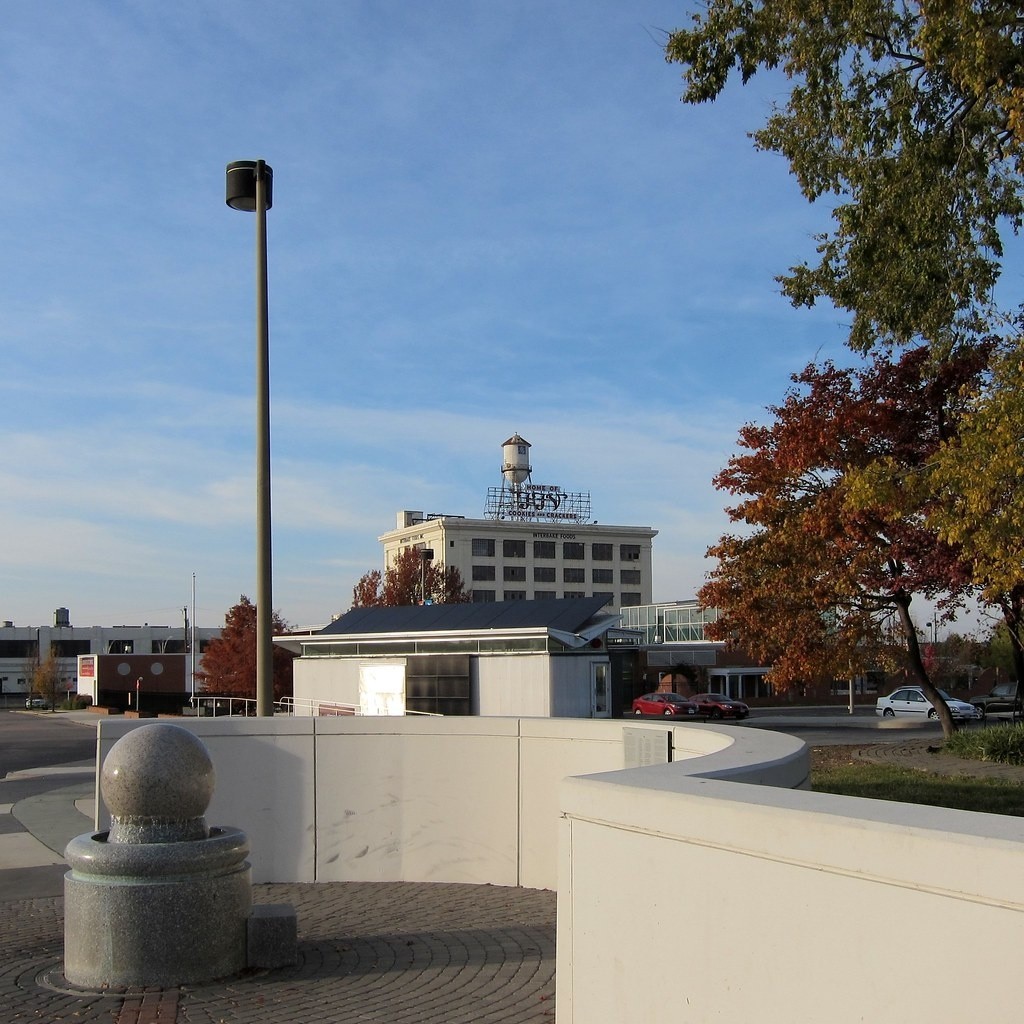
[875,686,975,721]
[688,692,750,719]
[891,686,963,702]
[633,692,699,715]
[26,695,46,710]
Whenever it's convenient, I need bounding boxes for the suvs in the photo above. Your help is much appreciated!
[969,682,1024,718]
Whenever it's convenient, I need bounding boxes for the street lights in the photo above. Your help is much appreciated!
[154,636,173,653]
[137,677,144,710]
[926,622,932,644]
[226,159,272,717]
[95,637,119,654]
